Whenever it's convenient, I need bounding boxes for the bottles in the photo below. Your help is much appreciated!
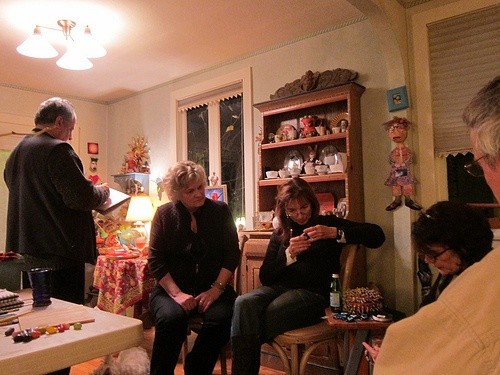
[330,274,342,314]
[318,118,347,136]
[284,149,303,177]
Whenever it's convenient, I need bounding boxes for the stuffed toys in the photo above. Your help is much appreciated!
[384,115,423,211]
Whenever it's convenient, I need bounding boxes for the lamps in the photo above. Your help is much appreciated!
[126,195,155,239]
[16,19,107,71]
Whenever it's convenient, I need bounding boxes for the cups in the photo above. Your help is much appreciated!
[28,267,52,307]
[135,233,148,253]
[274,134,281,142]
[278,170,290,178]
[265,170,278,179]
[259,212,273,229]
[304,163,344,175]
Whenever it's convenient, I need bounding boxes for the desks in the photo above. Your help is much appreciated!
[0,288,144,375]
[91,244,155,314]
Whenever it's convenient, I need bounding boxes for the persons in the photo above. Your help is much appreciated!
[230,178,386,375]
[140,160,240,375]
[6,97,108,375]
[372,81,500,375]
[362,201,476,363]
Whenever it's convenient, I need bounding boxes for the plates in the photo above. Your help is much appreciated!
[276,124,297,140]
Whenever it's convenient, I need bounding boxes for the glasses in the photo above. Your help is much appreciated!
[464,152,489,176]
[419,247,449,264]
[285,206,310,218]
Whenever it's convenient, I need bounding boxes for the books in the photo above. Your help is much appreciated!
[93,185,131,216]
[0,288,24,325]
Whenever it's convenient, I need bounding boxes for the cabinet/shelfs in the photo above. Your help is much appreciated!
[253,83,365,236]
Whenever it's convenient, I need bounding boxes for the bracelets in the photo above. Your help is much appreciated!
[214,281,226,290]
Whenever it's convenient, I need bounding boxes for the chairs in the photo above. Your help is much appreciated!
[264,245,357,375]
[180,318,228,375]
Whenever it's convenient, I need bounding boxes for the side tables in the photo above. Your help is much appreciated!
[323,307,407,375]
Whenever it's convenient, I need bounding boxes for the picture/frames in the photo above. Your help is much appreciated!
[387,85,408,111]
[205,186,228,204]
